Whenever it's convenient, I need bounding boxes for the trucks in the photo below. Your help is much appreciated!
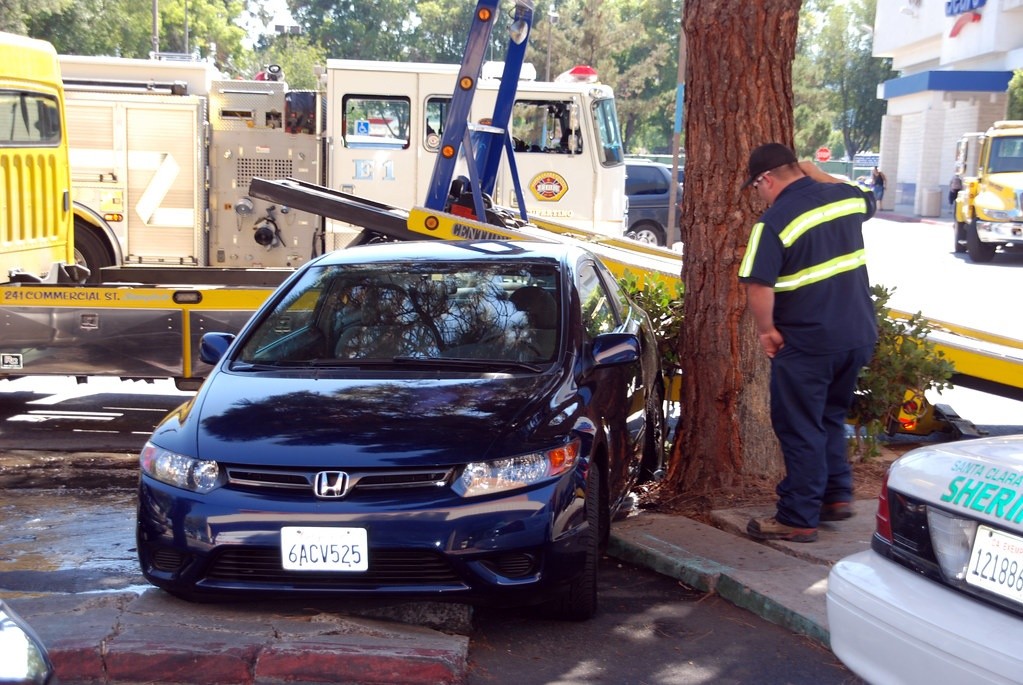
[947,119,1023,264]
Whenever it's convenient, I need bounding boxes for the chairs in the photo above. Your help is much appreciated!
[481,288,555,358]
[337,289,443,359]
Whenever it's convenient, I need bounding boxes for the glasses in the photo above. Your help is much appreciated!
[752,170,771,190]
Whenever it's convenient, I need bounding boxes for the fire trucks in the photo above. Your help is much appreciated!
[53,48,630,287]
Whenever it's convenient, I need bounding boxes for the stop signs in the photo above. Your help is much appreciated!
[816,148,831,162]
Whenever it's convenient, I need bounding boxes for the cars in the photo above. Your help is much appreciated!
[624,151,684,253]
[823,432,1023,685]
[132,235,667,623]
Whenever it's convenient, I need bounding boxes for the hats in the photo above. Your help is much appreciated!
[740,142,798,192]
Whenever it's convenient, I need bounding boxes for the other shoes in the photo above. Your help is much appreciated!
[746,516,818,543]
[819,501,858,521]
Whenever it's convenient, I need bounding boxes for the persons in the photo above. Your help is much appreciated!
[870,166,886,211]
[947,175,962,215]
[737,143,879,543]
